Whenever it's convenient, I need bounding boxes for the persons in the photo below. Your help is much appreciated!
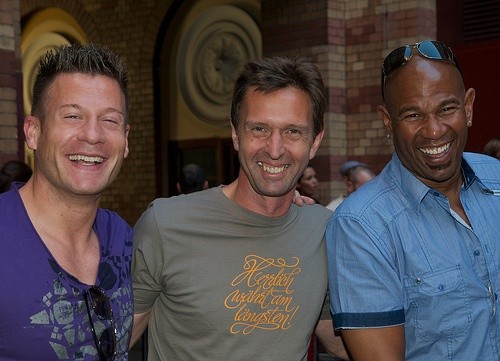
[299,159,377,212]
[176,163,208,194]
[128,57,350,361]
[325,40,500,361]
[0,45,315,361]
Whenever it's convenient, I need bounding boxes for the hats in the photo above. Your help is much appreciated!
[341,161,366,173]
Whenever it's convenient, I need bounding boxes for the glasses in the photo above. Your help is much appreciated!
[82,286,116,361]
[381,40,464,104]
